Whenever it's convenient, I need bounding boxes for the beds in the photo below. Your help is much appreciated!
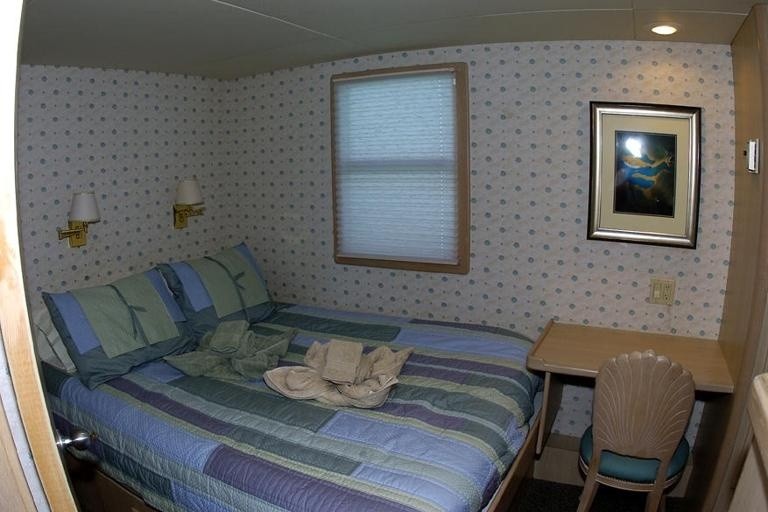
[28,240,537,512]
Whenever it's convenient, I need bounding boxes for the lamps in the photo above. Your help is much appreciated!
[58,190,103,250]
[172,178,206,229]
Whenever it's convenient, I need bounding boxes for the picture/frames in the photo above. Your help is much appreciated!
[585,99,704,252]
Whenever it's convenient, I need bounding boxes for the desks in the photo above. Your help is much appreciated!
[528,315,733,460]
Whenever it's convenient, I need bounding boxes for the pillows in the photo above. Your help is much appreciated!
[30,240,277,391]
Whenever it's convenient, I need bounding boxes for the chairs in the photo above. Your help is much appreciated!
[576,350,697,512]
